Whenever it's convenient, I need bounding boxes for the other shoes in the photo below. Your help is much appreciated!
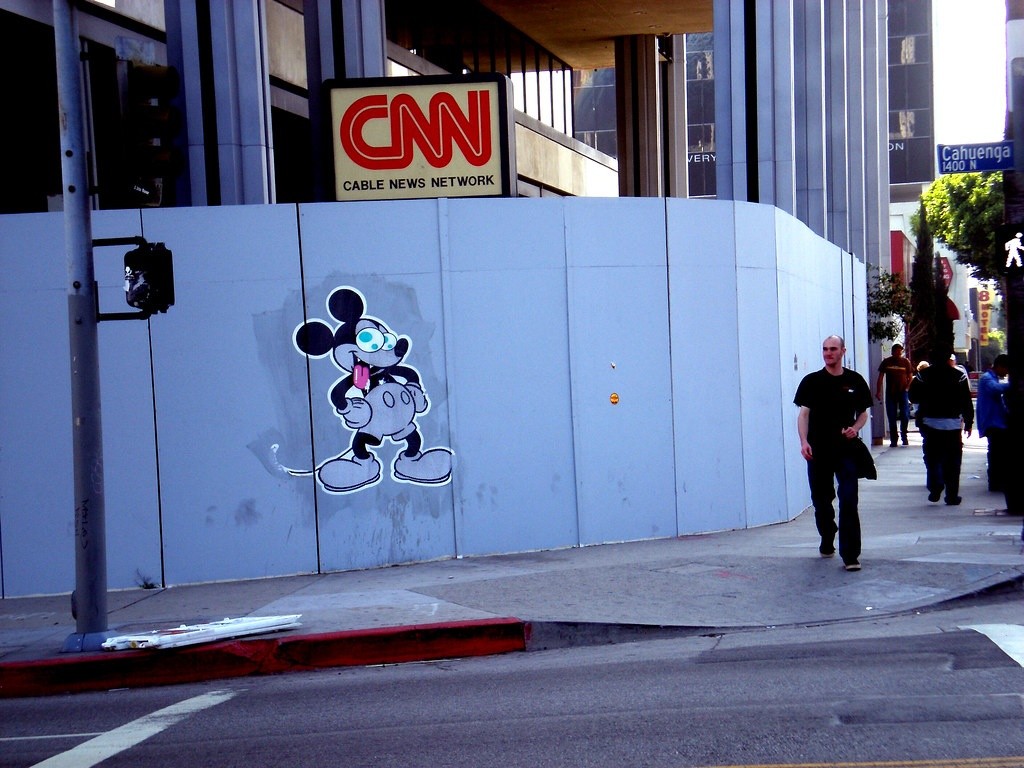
[890,444,897,447]
[928,490,940,502]
[819,534,834,557]
[902,441,908,445]
[843,559,861,569]
[948,496,962,505]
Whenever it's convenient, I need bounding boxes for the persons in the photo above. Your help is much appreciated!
[793,335,874,571]
[976,354,1013,492]
[875,343,913,447]
[908,339,974,506]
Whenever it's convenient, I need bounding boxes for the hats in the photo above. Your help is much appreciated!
[949,354,956,360]
